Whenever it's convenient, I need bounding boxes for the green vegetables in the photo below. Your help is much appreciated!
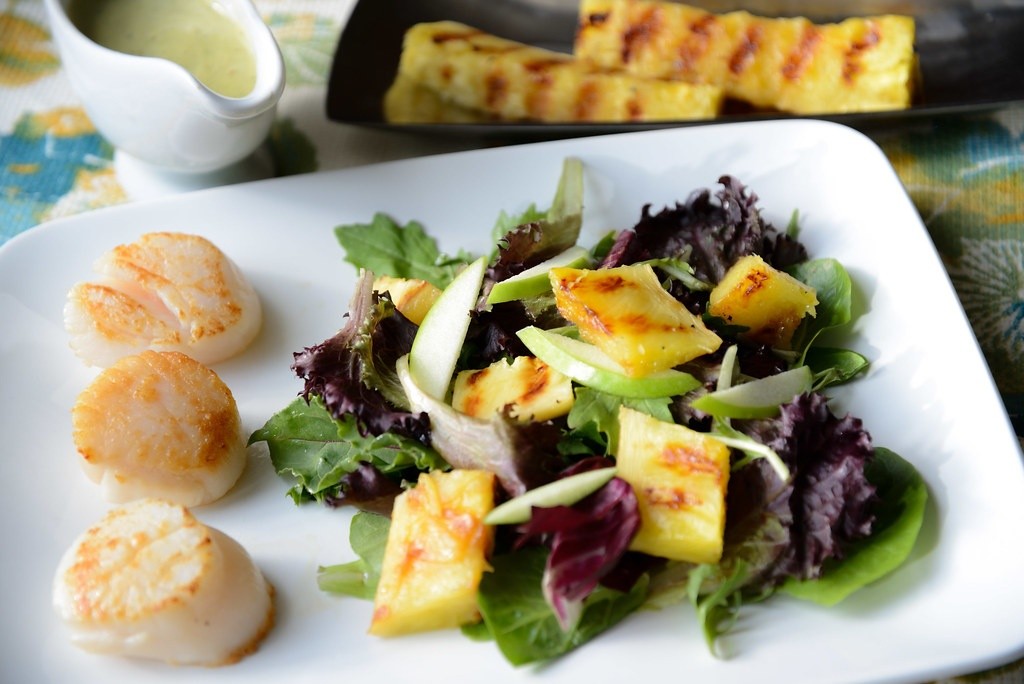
[250,159,927,664]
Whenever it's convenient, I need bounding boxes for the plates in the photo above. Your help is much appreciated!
[0,120,1024,683]
[322,0,1024,140]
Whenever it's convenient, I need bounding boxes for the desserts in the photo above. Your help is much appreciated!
[384,0,915,125]
